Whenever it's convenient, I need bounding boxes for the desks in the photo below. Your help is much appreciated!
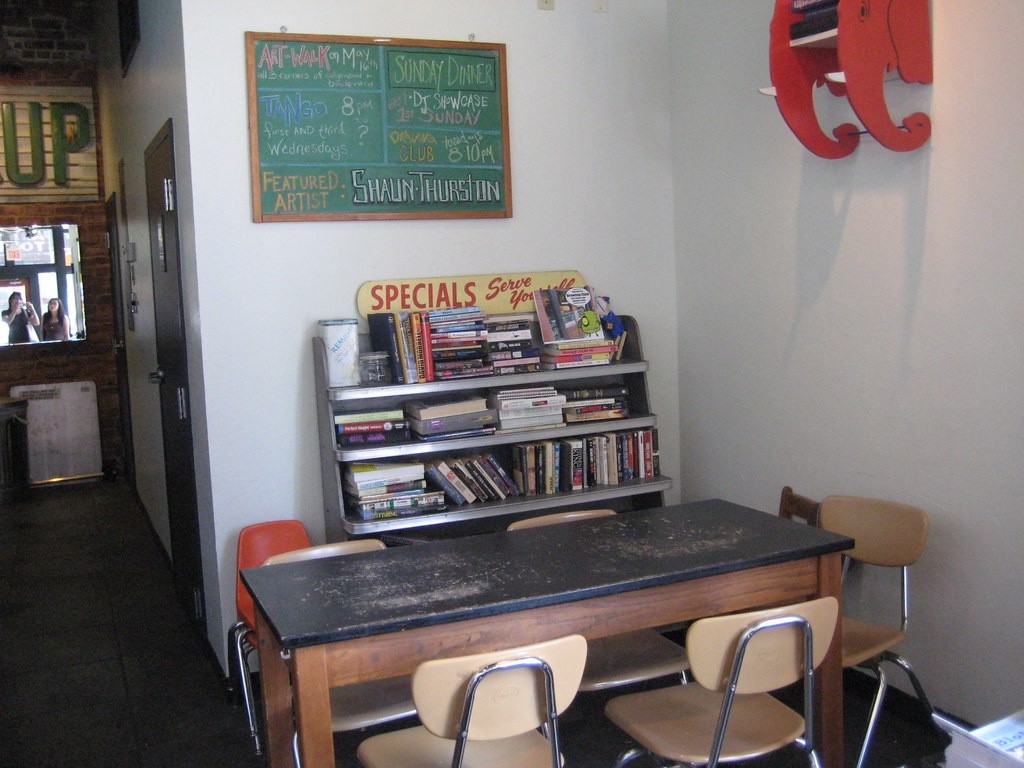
[239,496,856,768]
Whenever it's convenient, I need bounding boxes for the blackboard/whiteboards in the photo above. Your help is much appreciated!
[243,30,512,223]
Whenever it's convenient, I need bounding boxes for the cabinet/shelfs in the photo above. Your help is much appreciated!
[313,313,674,540]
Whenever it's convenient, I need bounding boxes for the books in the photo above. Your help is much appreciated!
[533,285,627,370]
[343,428,661,521]
[368,306,540,385]
[931,712,1024,768]
[380,534,431,544]
[333,384,631,448]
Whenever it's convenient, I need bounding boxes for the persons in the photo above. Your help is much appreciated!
[41,298,71,341]
[1,292,41,344]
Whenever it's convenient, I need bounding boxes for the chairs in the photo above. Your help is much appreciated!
[229,486,939,768]
